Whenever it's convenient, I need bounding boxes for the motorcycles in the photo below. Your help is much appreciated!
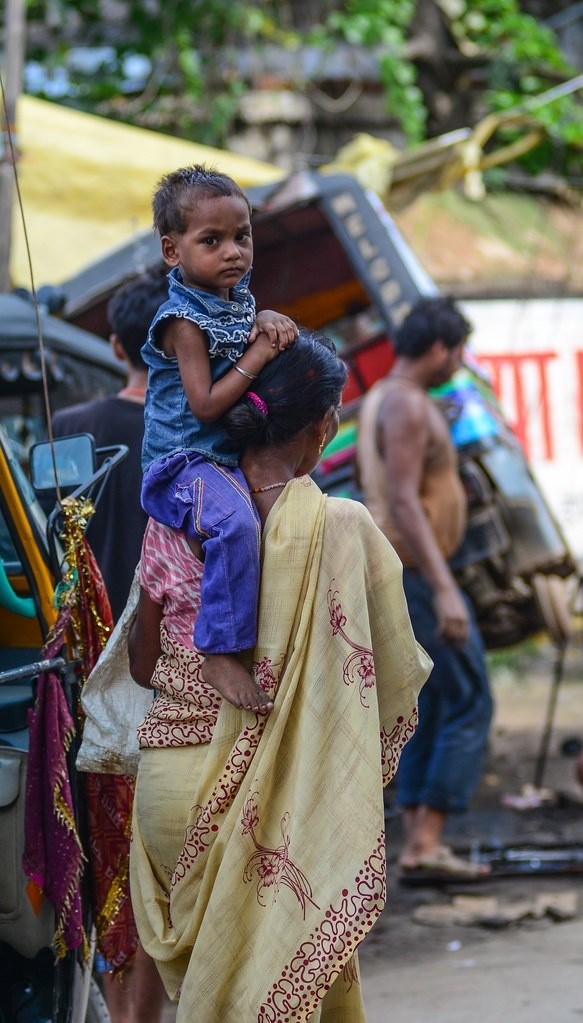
[0,431,131,1023]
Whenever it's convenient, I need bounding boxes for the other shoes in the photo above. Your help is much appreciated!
[397,848,489,887]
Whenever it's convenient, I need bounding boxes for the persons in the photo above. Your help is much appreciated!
[98,326,435,1023]
[355,294,494,883]
[141,159,300,711]
[36,271,169,627]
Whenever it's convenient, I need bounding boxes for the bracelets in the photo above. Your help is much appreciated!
[232,363,258,380]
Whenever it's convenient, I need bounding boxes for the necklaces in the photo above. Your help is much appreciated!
[250,482,286,495]
[117,387,149,397]
